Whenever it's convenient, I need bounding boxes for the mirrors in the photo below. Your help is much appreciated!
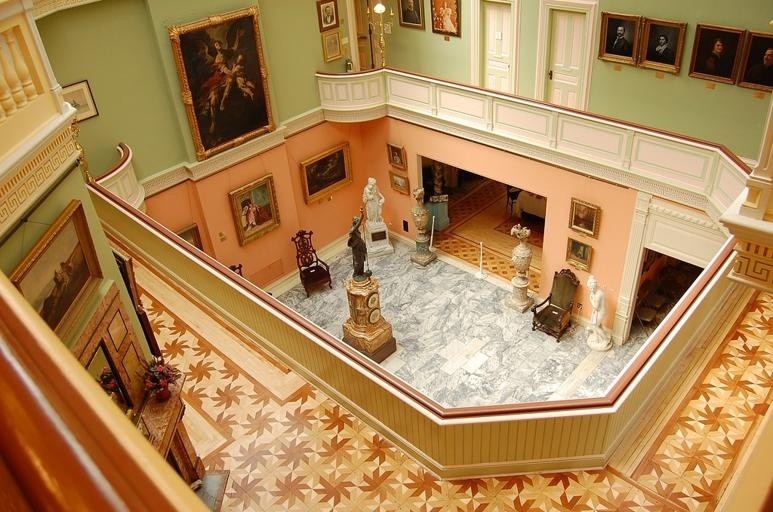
[85,338,134,414]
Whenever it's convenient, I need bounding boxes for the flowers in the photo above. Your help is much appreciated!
[98,367,120,395]
[144,358,178,394]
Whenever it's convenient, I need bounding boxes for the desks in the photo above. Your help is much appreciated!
[513,190,547,219]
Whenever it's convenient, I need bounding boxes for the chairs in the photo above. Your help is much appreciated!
[632,298,659,338]
[228,264,272,296]
[506,185,521,216]
[639,287,667,310]
[290,229,333,298]
[531,269,580,343]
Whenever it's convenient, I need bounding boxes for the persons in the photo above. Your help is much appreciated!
[323,5,334,25]
[705,38,726,74]
[654,34,672,58]
[363,175,385,229]
[347,206,367,282]
[402,0,420,24]
[240,204,260,232]
[187,29,256,134]
[439,1,454,29]
[746,48,773,84]
[611,25,629,51]
[586,274,610,347]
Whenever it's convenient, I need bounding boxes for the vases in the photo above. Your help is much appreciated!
[155,388,171,402]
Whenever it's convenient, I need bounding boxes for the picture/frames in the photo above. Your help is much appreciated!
[565,237,593,273]
[597,11,773,92]
[174,223,204,252]
[387,143,408,171]
[568,196,602,239]
[227,173,281,247]
[168,5,276,162]
[299,142,353,206]
[61,80,100,125]
[8,199,104,344]
[316,0,462,68]
[388,170,410,196]
